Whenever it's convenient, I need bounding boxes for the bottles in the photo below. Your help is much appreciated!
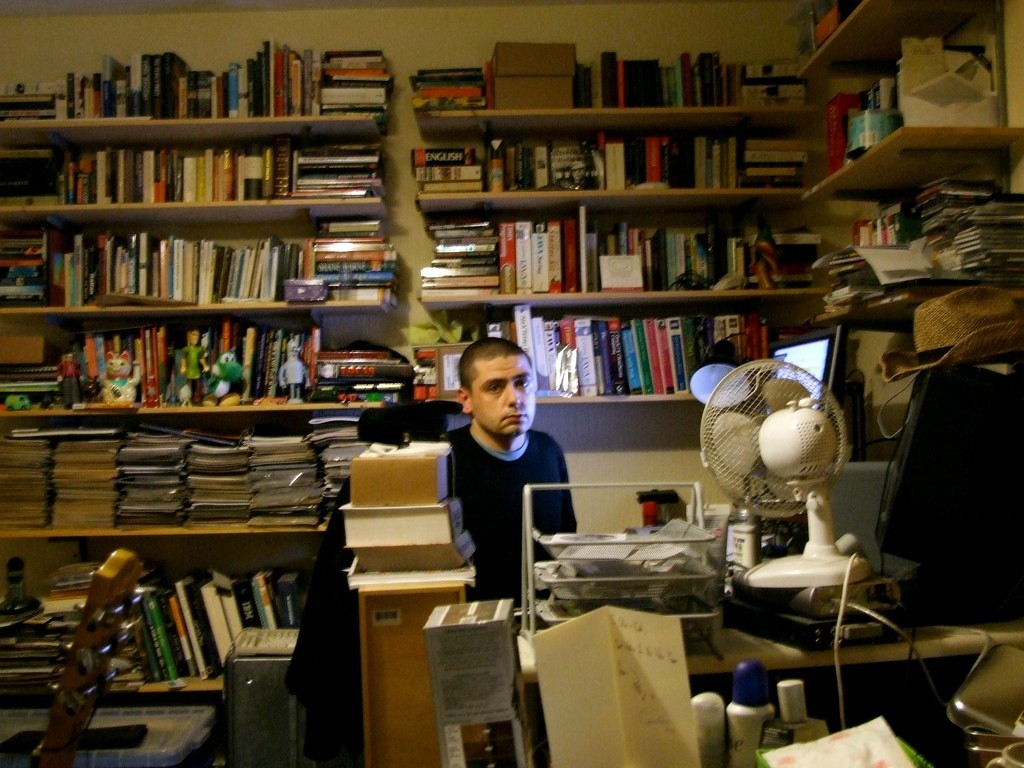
[726,510,754,593]
[689,660,830,768]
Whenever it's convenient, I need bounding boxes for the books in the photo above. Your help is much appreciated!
[0,35,1024,694]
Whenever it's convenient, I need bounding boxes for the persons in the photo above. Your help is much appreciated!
[56,352,84,409]
[278,340,309,404]
[436,337,576,606]
[179,330,208,402]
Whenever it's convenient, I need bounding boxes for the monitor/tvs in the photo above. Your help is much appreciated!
[768,324,845,417]
[873,363,1024,626]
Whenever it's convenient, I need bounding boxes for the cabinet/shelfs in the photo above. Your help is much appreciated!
[412,105,820,404]
[341,555,476,768]
[222,627,305,768]
[796,0,1024,341]
[0,114,386,693]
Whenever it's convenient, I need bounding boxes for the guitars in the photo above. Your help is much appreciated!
[32,549,146,768]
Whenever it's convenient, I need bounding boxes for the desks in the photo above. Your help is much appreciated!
[514,618,1024,768]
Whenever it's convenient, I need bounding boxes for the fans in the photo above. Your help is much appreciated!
[699,358,869,587]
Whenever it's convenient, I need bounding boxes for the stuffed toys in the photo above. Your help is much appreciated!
[203,348,242,407]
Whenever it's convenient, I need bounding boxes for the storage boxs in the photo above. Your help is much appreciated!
[0,706,216,768]
